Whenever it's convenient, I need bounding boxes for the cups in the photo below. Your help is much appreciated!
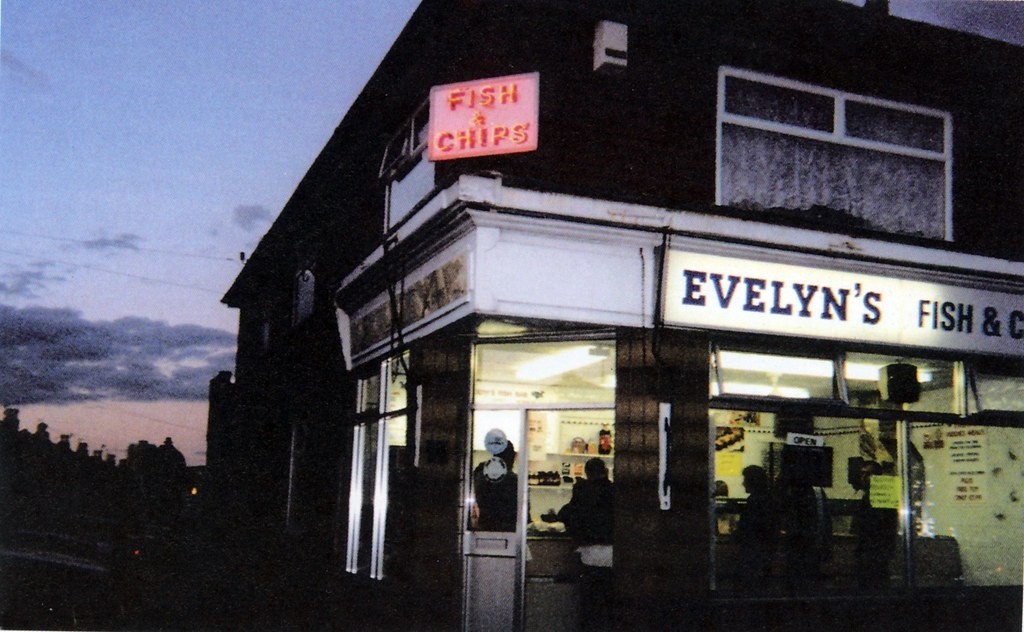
[528,470,561,486]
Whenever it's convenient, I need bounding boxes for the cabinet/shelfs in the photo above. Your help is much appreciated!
[528,451,614,492]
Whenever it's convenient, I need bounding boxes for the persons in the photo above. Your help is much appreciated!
[473,439,832,535]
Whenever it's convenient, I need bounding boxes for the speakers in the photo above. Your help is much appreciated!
[878,364,919,402]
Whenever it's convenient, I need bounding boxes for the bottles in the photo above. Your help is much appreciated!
[575,463,583,477]
[598,422,611,455]
[562,462,570,475]
